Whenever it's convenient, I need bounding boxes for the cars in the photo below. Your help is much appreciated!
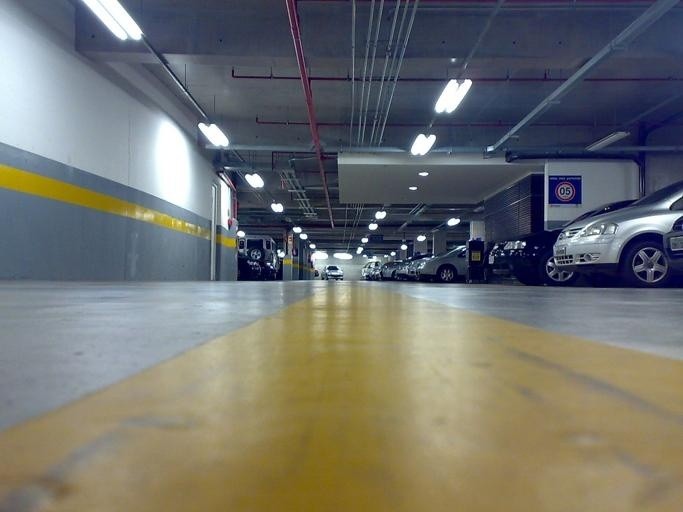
[321,265,344,281]
[361,245,466,283]
[238,234,280,281]
[488,179,683,288]
